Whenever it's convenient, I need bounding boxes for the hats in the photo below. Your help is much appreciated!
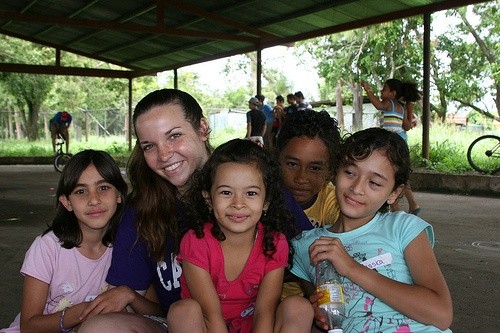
[249,98,258,105]
[255,95,265,99]
[61,112,68,121]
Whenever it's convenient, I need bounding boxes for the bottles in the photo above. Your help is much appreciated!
[315,260,345,333]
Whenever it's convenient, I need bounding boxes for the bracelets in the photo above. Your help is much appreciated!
[60,307,72,332]
[366,89,371,92]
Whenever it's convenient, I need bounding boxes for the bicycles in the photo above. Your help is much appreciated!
[467,134,500,175]
[53,141,72,173]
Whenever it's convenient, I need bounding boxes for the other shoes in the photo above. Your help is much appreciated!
[408,207,421,215]
[54,152,56,156]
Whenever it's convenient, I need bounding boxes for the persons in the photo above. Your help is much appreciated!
[0,149,165,333]
[287,127,454,333]
[272,108,341,333]
[77,89,315,333]
[245,91,312,155]
[361,79,421,215]
[48,111,73,157]
[167,139,315,333]
